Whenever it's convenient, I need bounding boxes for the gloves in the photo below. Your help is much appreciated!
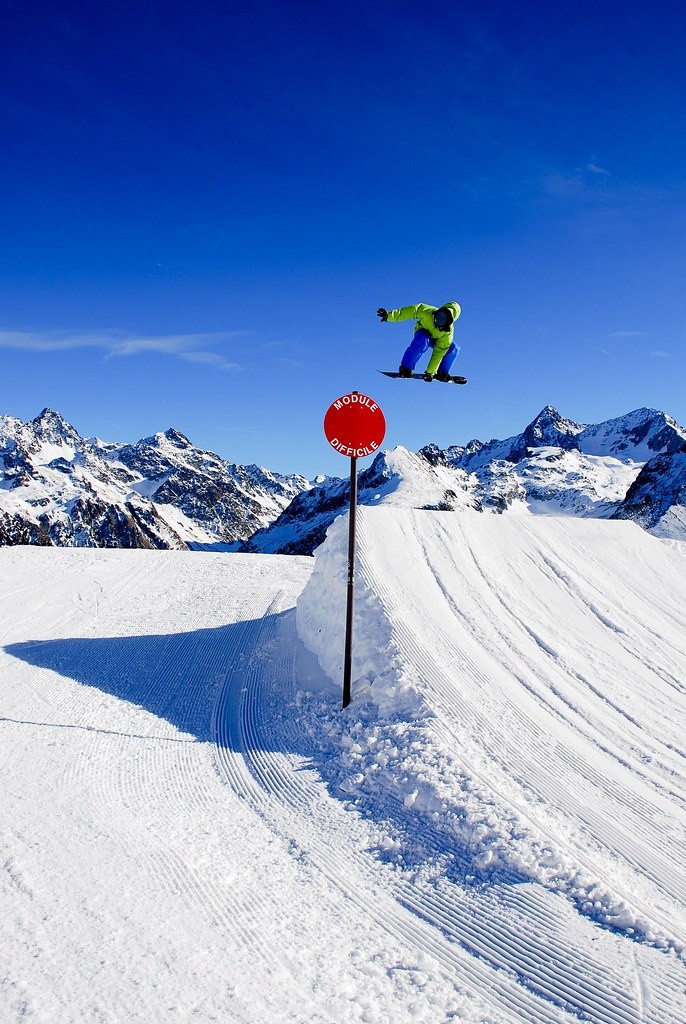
[423,374,433,382]
[376,307,389,323]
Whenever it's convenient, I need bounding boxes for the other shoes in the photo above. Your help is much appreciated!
[400,365,414,379]
[435,372,450,382]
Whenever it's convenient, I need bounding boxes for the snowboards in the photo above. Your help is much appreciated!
[376,368,468,385]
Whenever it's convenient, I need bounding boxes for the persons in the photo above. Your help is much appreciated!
[377,301,461,382]
